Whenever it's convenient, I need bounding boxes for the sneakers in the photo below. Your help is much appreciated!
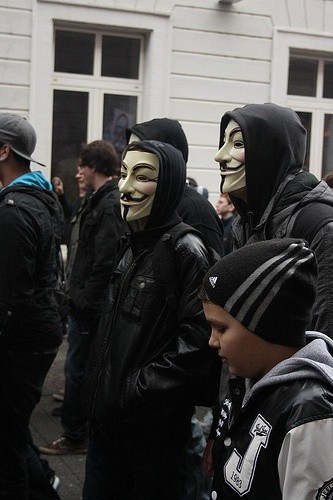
[38,436,87,454]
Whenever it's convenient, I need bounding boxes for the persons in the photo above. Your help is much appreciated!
[0,102,333,500]
[198,238,333,500]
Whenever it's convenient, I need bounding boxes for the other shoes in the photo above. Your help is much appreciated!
[51,406,63,417]
[49,475,61,490]
[52,388,65,401]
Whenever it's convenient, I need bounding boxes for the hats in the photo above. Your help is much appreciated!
[0,112,46,167]
[202,238,319,349]
